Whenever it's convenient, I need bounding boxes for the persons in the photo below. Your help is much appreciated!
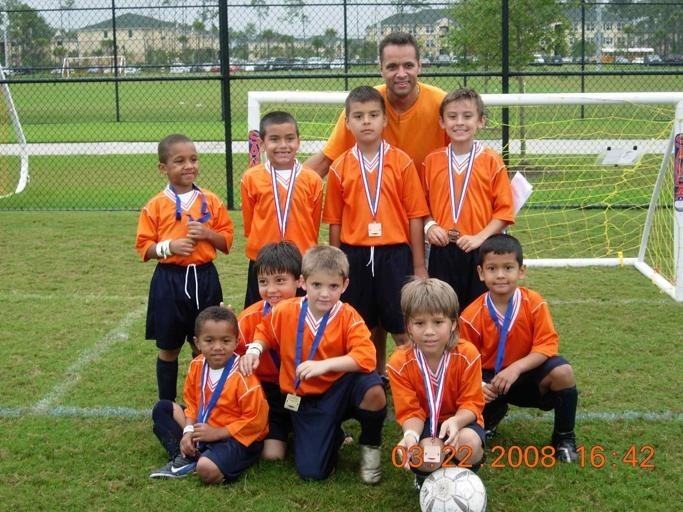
[133,133,235,403]
[238,243,388,486]
[149,305,270,485]
[302,32,451,189]
[418,87,517,317]
[458,232,580,465]
[321,85,433,395]
[383,277,488,490]
[241,110,323,310]
[234,238,302,462]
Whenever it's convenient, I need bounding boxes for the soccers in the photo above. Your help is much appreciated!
[420,466,488,512]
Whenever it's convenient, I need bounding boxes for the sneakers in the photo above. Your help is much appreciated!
[555,439,578,465]
[359,445,383,486]
[149,456,198,479]
[483,405,510,439]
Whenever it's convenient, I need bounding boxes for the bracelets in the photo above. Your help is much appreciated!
[367,222,382,238]
[181,425,194,435]
[156,239,174,260]
[481,382,487,388]
[423,220,437,236]
[403,430,420,443]
[244,342,263,358]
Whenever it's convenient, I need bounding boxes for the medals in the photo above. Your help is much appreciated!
[284,394,301,411]
[447,228,460,243]
[422,444,441,464]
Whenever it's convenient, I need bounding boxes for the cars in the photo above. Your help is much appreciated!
[169,56,352,75]
[88,66,137,76]
